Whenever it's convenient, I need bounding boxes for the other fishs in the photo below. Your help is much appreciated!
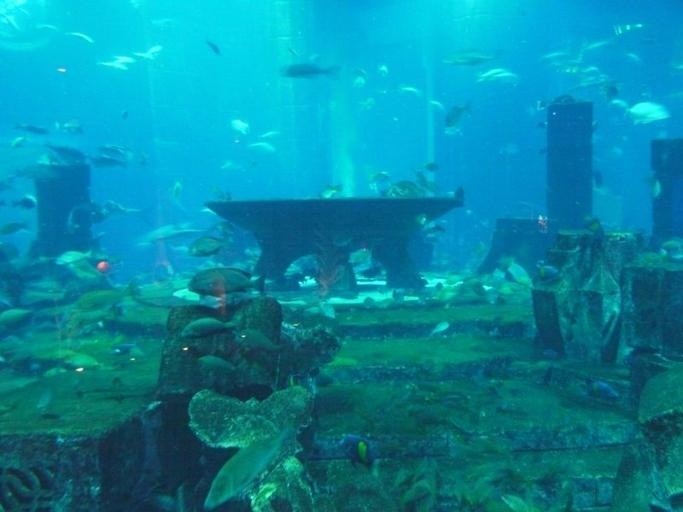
[0,0,683,512]
